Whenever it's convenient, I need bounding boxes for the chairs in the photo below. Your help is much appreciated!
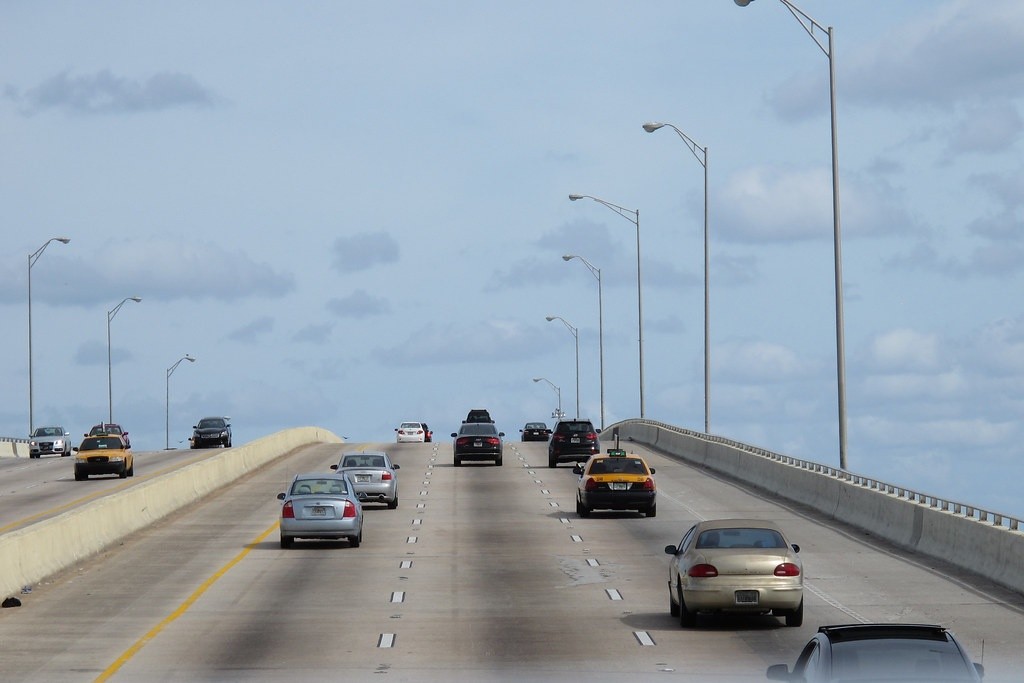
[298,486,311,494]
[329,486,340,493]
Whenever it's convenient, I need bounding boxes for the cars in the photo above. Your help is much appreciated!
[765,621,987,683]
[408,422,434,443]
[329,449,401,510]
[28,425,72,459]
[519,422,551,442]
[276,471,368,549]
[395,421,428,444]
[450,422,505,467]
[663,516,805,628]
[72,421,134,482]
[572,448,658,519]
[187,416,232,450]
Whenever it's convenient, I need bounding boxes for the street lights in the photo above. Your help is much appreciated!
[106,296,142,425]
[562,253,605,430]
[641,119,710,436]
[568,192,646,420]
[533,377,561,420]
[27,236,72,439]
[733,0,849,470]
[545,315,580,420]
[166,356,196,451]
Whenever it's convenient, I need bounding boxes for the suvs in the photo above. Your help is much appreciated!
[461,409,496,425]
[544,418,602,470]
